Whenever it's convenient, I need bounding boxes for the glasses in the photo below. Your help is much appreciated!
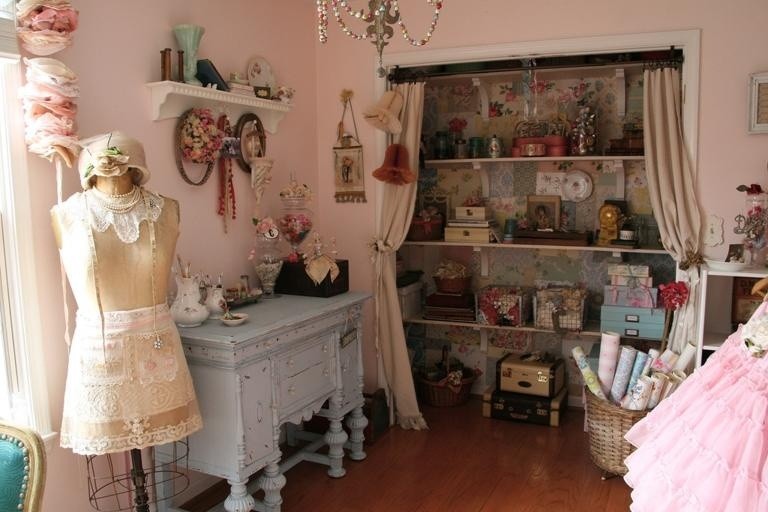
[317,0,442,81]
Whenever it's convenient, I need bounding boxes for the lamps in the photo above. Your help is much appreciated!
[0,424,47,511]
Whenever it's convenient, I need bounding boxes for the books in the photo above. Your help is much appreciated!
[397,281,423,321]
[604,284,659,308]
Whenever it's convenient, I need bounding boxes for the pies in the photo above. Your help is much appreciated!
[176,253,191,277]
[201,271,223,288]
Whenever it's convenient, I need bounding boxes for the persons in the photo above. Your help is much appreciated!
[48,131,201,458]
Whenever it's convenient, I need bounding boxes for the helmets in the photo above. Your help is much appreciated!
[561,170,594,203]
[703,257,749,272]
[247,56,274,89]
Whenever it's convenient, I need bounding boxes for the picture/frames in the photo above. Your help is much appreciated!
[153,289,374,512]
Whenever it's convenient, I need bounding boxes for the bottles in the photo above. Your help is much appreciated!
[76,137,150,190]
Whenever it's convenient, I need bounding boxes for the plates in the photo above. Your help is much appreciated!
[697,267,768,366]
[375,29,701,356]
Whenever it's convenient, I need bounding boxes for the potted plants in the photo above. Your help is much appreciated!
[746,72,768,133]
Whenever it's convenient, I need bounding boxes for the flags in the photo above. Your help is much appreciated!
[205,288,227,320]
[170,277,208,328]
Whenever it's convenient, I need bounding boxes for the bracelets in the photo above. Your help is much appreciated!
[220,312,249,327]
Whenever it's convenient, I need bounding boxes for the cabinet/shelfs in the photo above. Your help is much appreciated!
[435,130,449,160]
[454,138,469,159]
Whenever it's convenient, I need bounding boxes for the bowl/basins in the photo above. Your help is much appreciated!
[92,183,141,213]
